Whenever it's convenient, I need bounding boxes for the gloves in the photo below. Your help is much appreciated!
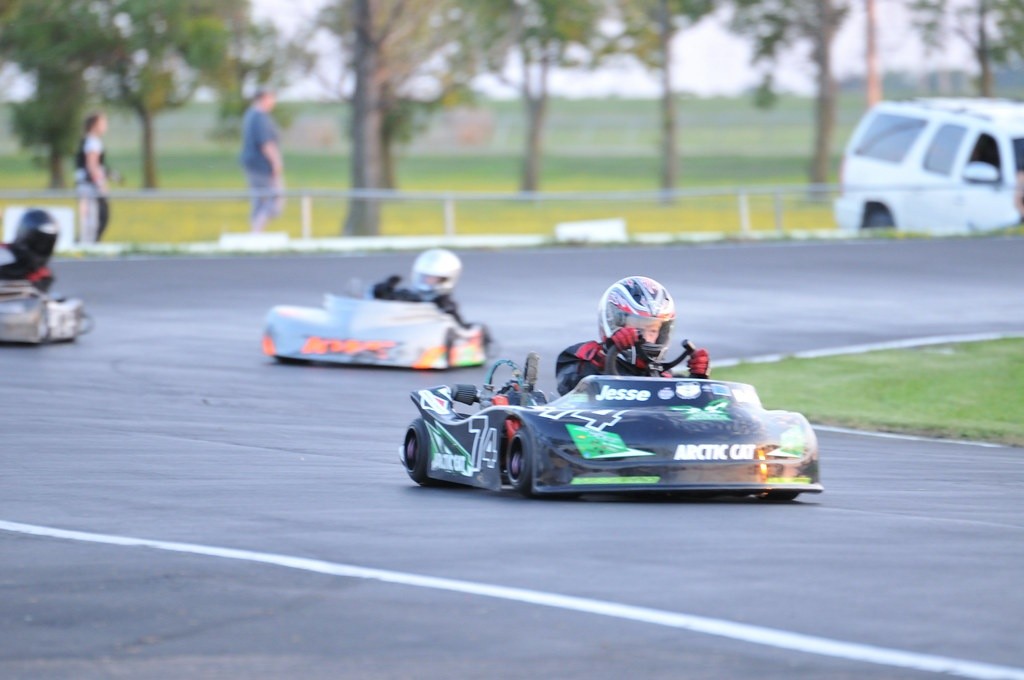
[611,327,638,352]
[383,275,402,295]
[687,348,709,378]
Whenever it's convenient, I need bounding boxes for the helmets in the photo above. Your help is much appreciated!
[597,275,676,364]
[412,250,461,301]
[16,210,58,256]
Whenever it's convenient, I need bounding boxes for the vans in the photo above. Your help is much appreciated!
[834,95,1024,237]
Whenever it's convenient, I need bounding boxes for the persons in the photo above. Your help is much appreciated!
[556,276,711,398]
[372,249,463,326]
[240,87,286,232]
[0,209,59,293]
[73,111,112,242]
